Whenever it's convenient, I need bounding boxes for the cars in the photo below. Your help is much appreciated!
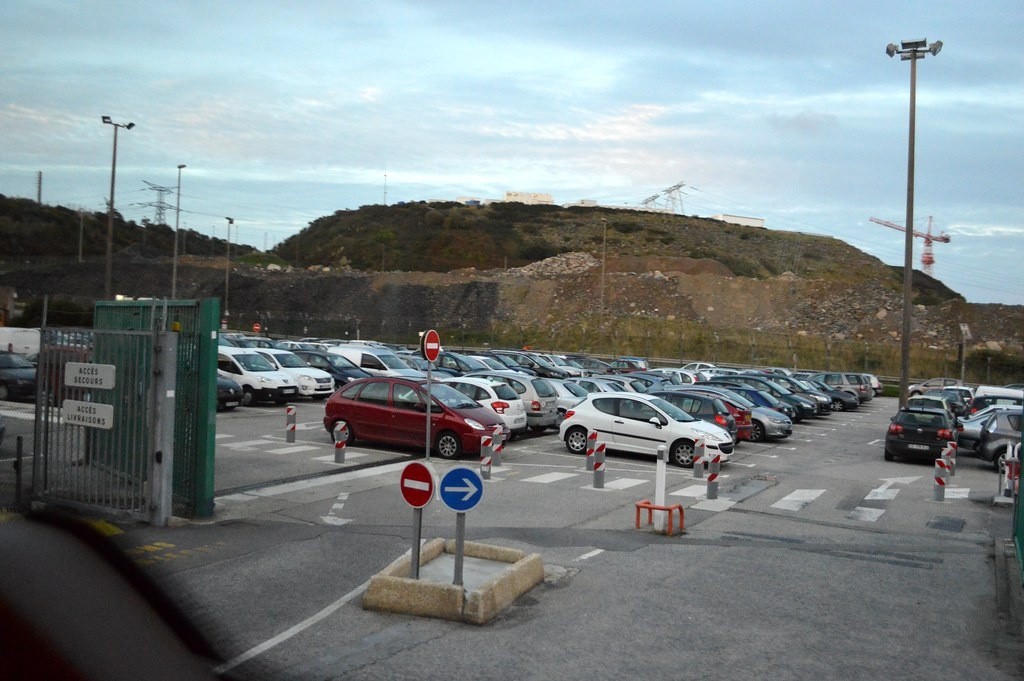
[909,376,963,395]
[0,349,37,401]
[26,331,883,447]
[323,376,512,460]
[884,384,1024,470]
[557,391,735,468]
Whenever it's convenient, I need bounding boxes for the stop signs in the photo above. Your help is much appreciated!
[399,462,435,509]
[253,323,261,331]
[421,329,440,364]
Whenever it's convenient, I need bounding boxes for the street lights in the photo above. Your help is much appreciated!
[100,114,136,301]
[171,163,187,299]
[221,216,235,328]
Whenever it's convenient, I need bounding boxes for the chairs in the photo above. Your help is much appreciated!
[631,401,645,417]
[905,414,917,423]
[932,417,943,425]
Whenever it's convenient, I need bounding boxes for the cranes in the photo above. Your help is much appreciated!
[867,215,952,277]
